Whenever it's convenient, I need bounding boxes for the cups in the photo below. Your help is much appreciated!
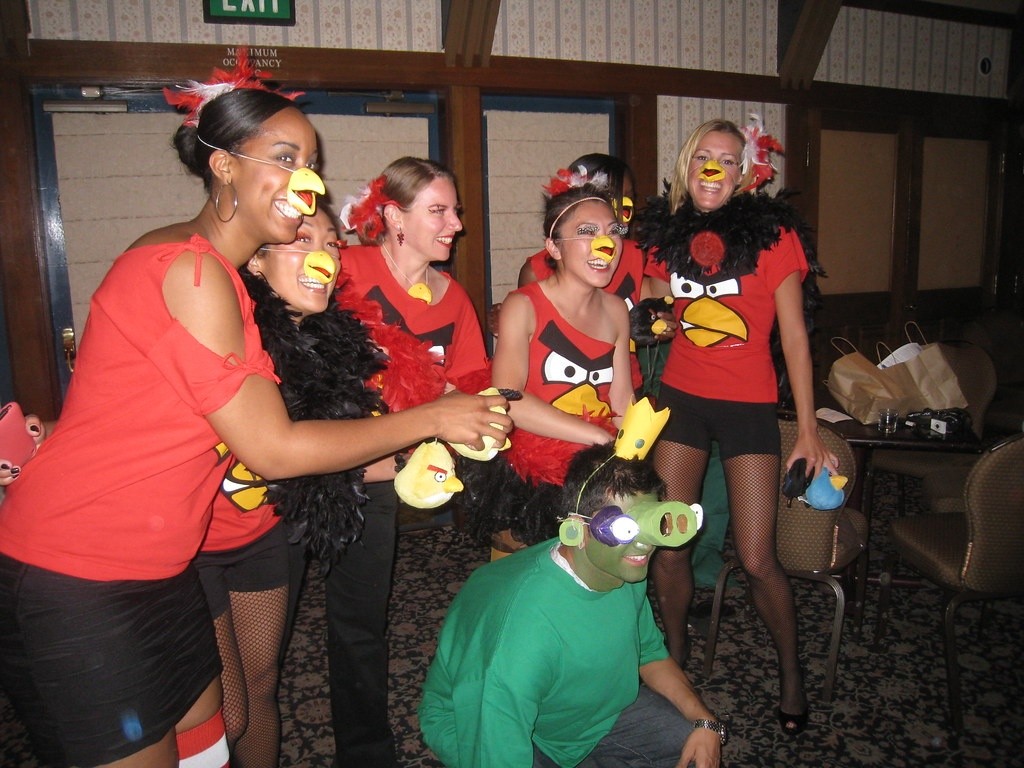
[877,408,898,434]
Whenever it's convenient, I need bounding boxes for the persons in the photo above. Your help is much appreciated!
[519,153,663,432]
[419,441,729,767]
[3,82,512,767]
[487,181,643,567]
[645,116,837,733]
[1,196,343,763]
[275,158,498,765]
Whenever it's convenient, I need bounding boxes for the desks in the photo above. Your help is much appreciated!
[784,384,1022,647]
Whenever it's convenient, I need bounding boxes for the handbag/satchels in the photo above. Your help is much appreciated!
[822,321,970,428]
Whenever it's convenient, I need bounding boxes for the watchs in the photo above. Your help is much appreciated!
[693,718,728,746]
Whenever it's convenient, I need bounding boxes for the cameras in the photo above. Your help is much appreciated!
[934,407,972,437]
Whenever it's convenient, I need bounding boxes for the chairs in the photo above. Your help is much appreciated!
[702,405,870,705]
[879,433,1023,725]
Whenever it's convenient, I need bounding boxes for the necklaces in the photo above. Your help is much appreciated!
[381,243,433,307]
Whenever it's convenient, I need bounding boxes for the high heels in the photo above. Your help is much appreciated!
[778,665,810,734]
[680,638,693,670]
